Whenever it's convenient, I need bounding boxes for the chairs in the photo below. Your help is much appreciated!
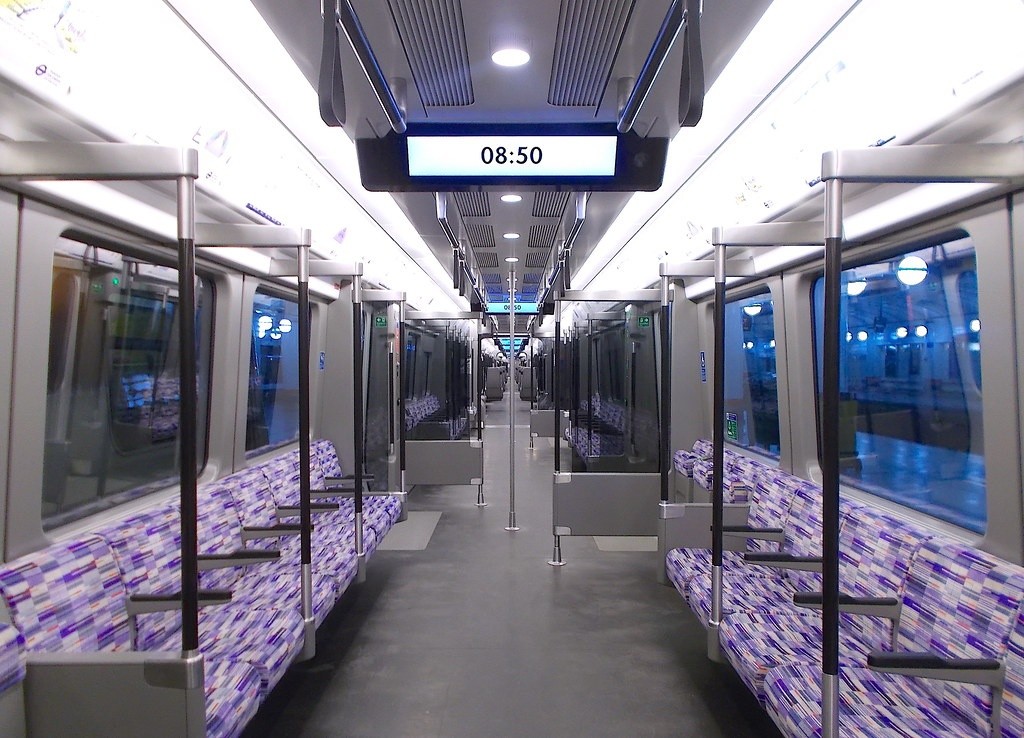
[0,437,401,738]
[564,399,627,460]
[664,438,1024,738]
[405,395,466,437]
